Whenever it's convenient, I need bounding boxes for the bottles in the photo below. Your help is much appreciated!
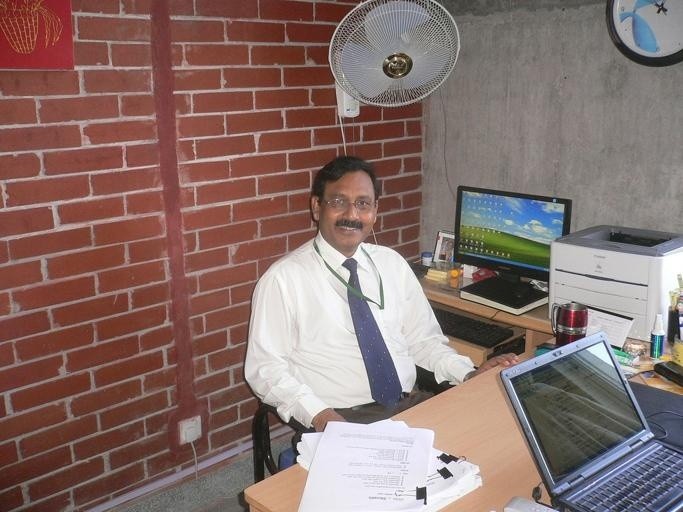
[421,251,432,266]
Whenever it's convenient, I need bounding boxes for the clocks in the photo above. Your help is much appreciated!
[605,0,682,67]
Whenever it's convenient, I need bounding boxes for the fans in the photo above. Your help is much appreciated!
[326,0,460,121]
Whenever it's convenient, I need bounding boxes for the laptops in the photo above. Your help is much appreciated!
[500,332,683,512]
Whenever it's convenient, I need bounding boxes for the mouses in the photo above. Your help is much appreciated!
[515,286,532,299]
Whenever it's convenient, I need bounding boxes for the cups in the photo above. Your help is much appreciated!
[550,302,587,349]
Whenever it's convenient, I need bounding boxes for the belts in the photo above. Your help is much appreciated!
[334,392,409,412]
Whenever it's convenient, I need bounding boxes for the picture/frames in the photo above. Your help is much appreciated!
[431,229,455,263]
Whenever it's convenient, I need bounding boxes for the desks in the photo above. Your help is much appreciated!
[396,258,683,364]
[243,336,682,512]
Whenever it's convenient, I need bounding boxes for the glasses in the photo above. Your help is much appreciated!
[323,199,375,210]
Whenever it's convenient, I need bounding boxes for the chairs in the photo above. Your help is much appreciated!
[250,365,455,483]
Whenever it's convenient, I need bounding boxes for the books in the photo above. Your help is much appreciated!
[424,262,461,282]
[458,275,549,316]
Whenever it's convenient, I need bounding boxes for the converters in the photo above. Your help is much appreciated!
[184,427,198,443]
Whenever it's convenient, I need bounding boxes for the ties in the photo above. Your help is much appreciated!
[342,258,402,411]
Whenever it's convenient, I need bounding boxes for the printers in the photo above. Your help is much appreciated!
[549,225,683,342]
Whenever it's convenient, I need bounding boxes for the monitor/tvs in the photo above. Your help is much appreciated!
[453,185,572,281]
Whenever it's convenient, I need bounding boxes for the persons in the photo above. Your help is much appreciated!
[243,156,519,458]
[441,241,454,261]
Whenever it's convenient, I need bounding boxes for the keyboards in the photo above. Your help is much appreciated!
[417,308,514,347]
